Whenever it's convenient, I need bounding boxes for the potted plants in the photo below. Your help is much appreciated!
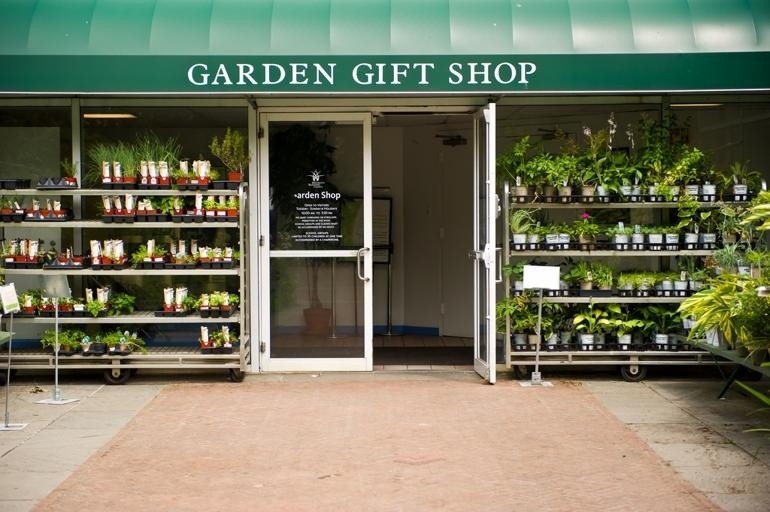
[4,286,111,318]
[198,325,237,353]
[40,326,148,356]
[154,284,240,318]
[496,110,770,368]
[1,178,88,270]
[279,161,347,336]
[84,125,252,271]
